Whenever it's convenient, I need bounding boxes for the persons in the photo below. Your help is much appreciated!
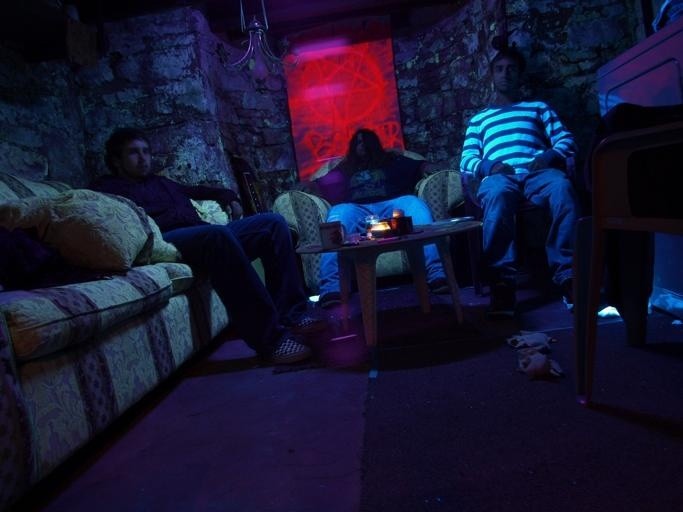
[301,127,447,310]
[101,124,326,364]
[457,45,578,311]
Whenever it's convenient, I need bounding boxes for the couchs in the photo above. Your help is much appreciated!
[1,171,265,512]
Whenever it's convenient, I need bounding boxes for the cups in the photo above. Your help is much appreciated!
[396,216,413,235]
[317,221,346,250]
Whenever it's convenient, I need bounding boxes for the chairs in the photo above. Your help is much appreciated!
[470,177,551,297]
[271,150,466,295]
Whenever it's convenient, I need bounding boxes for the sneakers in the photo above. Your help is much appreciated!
[427,276,451,293]
[289,316,333,337]
[486,285,518,317]
[561,283,574,310]
[320,289,344,310]
[255,336,317,364]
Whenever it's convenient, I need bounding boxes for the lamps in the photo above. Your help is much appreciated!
[224,1,299,97]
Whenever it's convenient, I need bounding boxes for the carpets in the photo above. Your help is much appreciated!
[364,313,683,511]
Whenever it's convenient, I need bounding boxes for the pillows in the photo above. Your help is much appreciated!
[146,213,184,262]
[1,189,154,269]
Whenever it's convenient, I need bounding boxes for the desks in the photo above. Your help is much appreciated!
[571,120,683,436]
